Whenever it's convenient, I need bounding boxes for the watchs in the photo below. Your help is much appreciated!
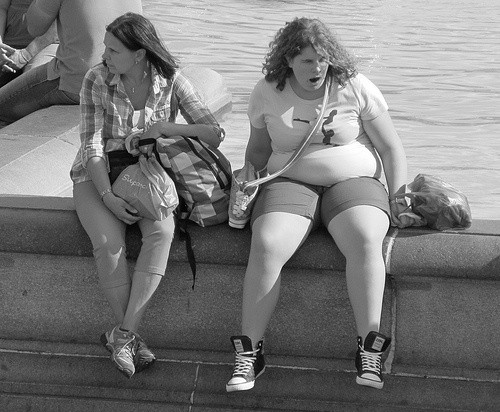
[390,198,407,207]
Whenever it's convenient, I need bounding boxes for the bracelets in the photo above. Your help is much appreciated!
[100,189,112,202]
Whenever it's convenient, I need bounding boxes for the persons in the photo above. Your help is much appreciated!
[70,11,226,378]
[0,0,143,129]
[224,16,420,391]
[0,0,59,87]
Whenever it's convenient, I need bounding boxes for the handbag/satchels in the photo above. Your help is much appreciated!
[390,174,472,231]
[112,132,232,228]
[228,168,260,229]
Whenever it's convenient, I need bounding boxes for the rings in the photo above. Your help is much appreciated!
[144,146,148,150]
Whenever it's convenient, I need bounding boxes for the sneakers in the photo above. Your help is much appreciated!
[354,331,392,390]
[100,325,156,379]
[226,334,267,392]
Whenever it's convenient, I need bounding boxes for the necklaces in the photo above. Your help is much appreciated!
[124,71,148,94]
